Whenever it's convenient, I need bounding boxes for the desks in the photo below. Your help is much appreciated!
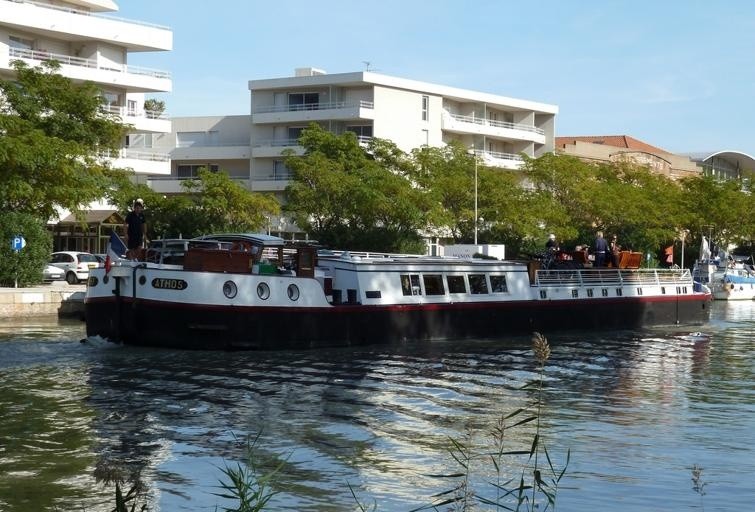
[151,238,232,264]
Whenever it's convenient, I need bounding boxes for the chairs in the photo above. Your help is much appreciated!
[543,250,643,273]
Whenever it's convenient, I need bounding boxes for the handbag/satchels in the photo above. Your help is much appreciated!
[606,246,610,257]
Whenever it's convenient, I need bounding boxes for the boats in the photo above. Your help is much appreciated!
[693,235,755,300]
[84,233,713,348]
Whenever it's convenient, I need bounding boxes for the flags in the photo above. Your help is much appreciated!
[667,255,672,263]
[666,245,672,254]
[104,230,127,275]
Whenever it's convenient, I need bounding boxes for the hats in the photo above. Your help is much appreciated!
[582,244,590,250]
[549,234,555,239]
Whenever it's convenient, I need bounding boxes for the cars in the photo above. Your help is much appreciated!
[43,251,126,284]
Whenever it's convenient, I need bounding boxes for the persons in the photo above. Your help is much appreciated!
[545,230,623,273]
[124,201,149,262]
